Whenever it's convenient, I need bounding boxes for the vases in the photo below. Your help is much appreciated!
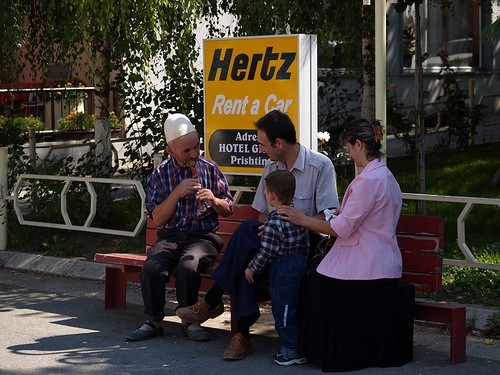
[30,129,120,142]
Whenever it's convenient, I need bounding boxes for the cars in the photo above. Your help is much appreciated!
[421,37,475,67]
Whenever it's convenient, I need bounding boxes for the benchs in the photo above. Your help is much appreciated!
[95,211,468,366]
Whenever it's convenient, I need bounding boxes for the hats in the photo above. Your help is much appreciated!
[164,113,196,144]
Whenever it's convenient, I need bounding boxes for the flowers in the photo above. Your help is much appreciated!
[55,110,121,131]
[54,82,88,107]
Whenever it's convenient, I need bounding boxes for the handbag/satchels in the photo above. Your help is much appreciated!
[308,237,335,281]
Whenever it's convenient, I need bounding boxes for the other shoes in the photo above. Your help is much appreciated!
[273,351,306,366]
[223,333,251,360]
[183,322,213,342]
[176,295,224,323]
[126,322,164,341]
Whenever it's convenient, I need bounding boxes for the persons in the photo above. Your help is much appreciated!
[127,113,234,340]
[245,169,312,366]
[175,110,339,360]
[278,119,402,280]
